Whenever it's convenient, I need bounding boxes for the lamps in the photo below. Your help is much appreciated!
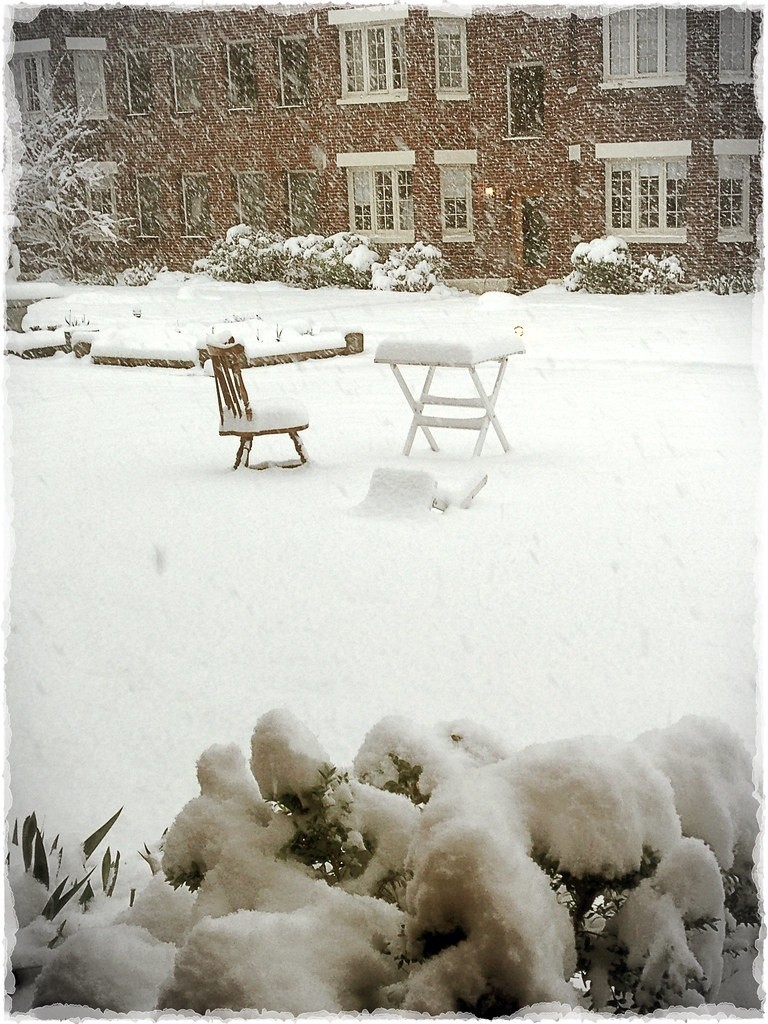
[485,184,496,201]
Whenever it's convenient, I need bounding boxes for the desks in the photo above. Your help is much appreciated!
[373,347,528,458]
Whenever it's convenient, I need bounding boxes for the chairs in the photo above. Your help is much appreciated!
[199,334,311,472]
